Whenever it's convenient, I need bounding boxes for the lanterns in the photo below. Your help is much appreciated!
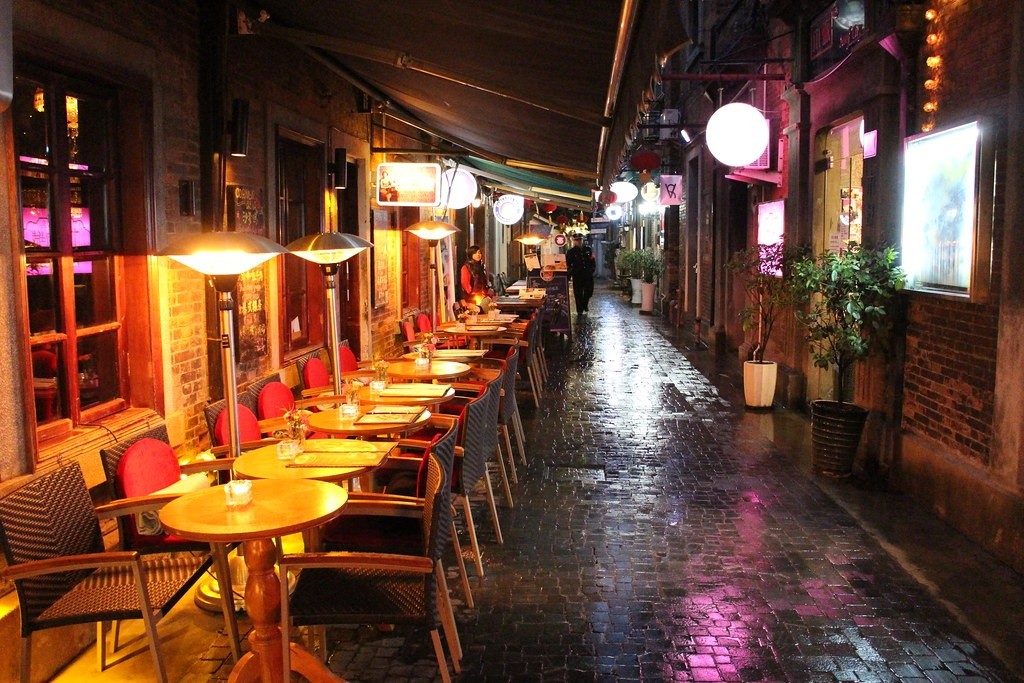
[543,201,556,225]
[557,214,567,226]
[630,150,660,184]
[598,189,617,206]
[575,212,589,224]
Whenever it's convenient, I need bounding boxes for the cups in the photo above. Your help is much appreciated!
[82,366,95,379]
[224,479,252,506]
[456,323,466,332]
[415,358,429,369]
[369,381,386,394]
[277,439,300,459]
[338,403,358,420]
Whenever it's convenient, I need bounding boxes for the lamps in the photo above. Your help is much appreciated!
[403,220,462,332]
[290,231,375,406]
[328,146,347,192]
[226,97,250,160]
[149,230,292,453]
[623,220,634,233]
[513,233,547,286]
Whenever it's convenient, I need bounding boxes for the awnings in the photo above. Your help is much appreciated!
[217,0,693,208]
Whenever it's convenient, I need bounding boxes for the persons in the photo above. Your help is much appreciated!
[567,233,597,317]
[379,172,401,201]
[460,246,495,303]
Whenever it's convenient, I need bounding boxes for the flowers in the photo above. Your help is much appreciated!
[282,407,312,444]
[421,333,438,350]
[418,342,435,361]
[373,359,390,379]
[344,381,364,403]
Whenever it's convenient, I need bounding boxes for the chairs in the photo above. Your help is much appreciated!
[498,272,508,294]
[0,300,550,683]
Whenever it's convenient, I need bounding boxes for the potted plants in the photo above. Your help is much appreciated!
[788,240,909,478]
[725,243,817,410]
[616,247,665,313]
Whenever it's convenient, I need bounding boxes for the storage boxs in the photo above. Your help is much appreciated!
[518,287,547,299]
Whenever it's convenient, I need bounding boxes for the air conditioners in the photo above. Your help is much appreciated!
[659,109,679,138]
[641,110,661,139]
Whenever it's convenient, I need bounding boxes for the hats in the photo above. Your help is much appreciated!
[573,233,583,239]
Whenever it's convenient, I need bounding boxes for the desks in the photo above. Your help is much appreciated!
[513,279,527,285]
[477,313,520,319]
[499,297,545,347]
[403,349,473,364]
[307,405,433,492]
[389,359,471,384]
[163,478,350,683]
[232,438,389,550]
[506,285,526,294]
[445,325,507,349]
[456,318,515,326]
[357,382,457,406]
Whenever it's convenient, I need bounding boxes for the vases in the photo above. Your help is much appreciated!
[346,393,362,415]
[419,351,433,368]
[377,370,389,390]
[289,423,307,451]
